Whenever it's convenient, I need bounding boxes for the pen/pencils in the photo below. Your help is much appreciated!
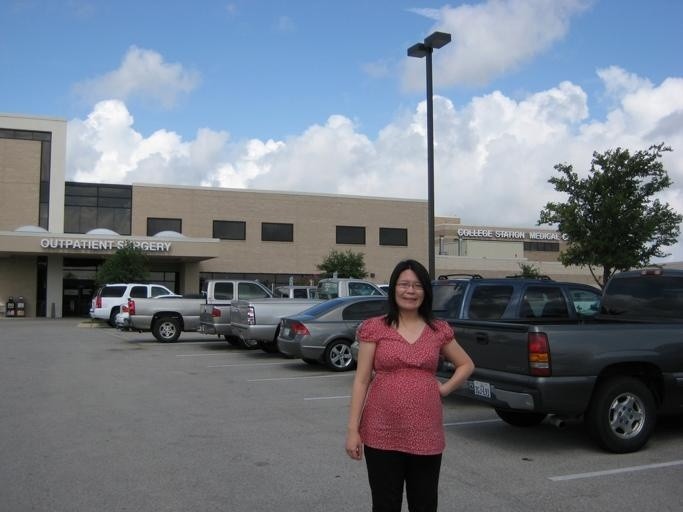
[90,283,176,329]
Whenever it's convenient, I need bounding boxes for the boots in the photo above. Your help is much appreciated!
[427,274,604,380]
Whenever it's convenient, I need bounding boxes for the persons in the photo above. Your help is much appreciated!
[344,258,474,512]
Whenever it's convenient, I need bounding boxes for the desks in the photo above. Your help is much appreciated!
[405,29,452,314]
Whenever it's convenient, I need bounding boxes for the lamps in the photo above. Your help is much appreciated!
[497,298,576,318]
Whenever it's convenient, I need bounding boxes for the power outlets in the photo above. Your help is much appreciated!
[115,279,389,373]
[438,268,683,454]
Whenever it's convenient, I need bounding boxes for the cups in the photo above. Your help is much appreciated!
[396,282,424,288]
